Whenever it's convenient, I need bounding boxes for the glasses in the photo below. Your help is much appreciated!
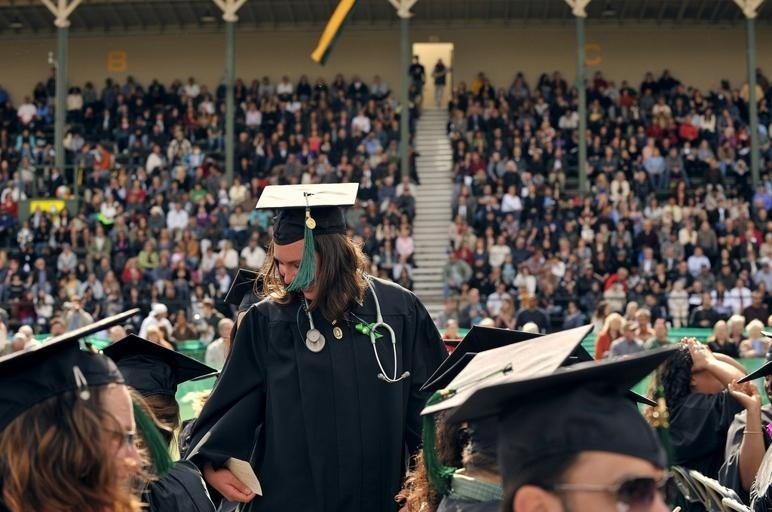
[560,471,680,508]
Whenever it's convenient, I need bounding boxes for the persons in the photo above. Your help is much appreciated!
[0,51,771,512]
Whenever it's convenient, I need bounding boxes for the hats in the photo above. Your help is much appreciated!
[417,324,688,487]
[0,308,139,435]
[224,268,282,315]
[761,331,772,362]
[255,183,364,295]
[99,333,221,397]
